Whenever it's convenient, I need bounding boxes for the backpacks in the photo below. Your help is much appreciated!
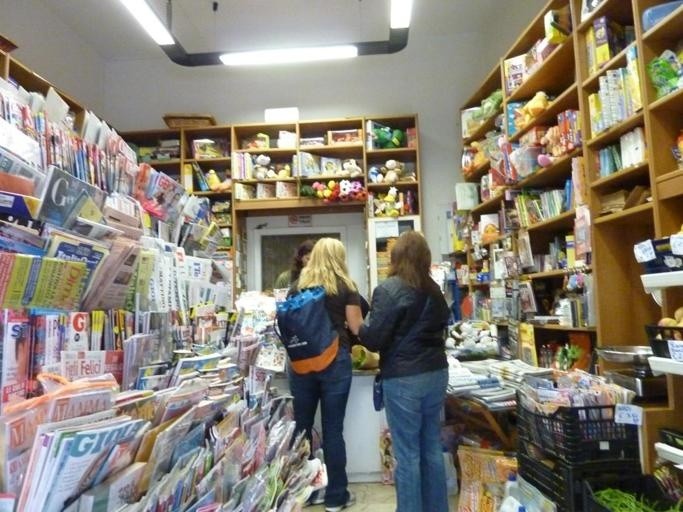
[273,286,339,374]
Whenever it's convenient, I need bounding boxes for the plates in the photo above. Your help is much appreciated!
[594,345,655,365]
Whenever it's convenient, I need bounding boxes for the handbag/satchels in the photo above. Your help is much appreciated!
[372,282,434,409]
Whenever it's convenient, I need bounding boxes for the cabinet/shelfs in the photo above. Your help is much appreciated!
[457,0,682,476]
[119,112,425,310]
[0,33,87,134]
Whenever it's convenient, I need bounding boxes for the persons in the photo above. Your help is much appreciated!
[359,233,449,511]
[285,238,371,512]
[276,241,316,288]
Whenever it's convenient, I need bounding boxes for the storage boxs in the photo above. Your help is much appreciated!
[518,475,558,511]
[517,436,642,511]
[515,387,641,466]
[582,472,678,512]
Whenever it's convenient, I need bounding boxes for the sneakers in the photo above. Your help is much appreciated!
[325,485,356,511]
[311,487,326,505]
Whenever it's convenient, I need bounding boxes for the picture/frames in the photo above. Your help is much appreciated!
[252,225,346,293]
